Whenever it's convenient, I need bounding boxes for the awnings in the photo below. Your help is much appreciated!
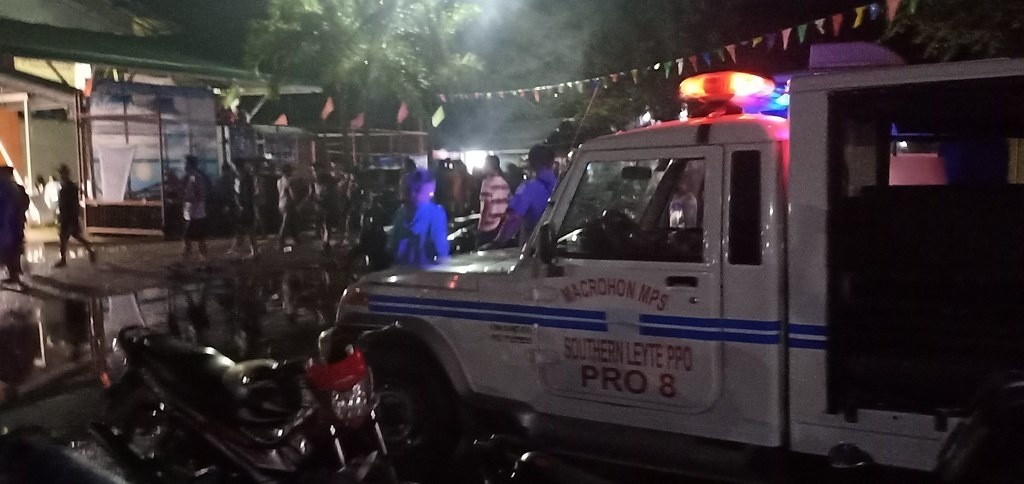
[0,17,323,124]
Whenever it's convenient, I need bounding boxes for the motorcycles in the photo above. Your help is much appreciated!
[100,280,429,484]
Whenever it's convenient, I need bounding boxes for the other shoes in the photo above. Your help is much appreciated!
[224,250,241,258]
[55,261,66,268]
[90,250,96,263]
[242,251,260,262]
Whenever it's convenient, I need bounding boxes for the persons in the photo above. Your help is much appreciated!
[0,163,97,284]
[670,173,697,233]
[180,145,558,269]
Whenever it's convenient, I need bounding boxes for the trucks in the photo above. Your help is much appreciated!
[316,42,1024,484]
